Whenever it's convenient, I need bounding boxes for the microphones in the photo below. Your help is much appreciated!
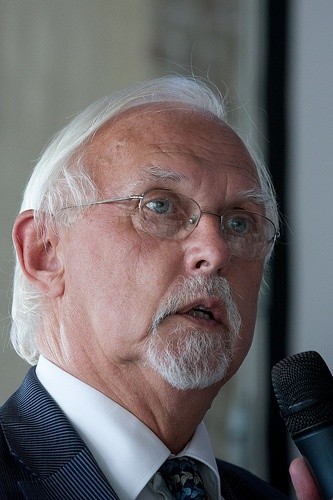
[272,351,333,500]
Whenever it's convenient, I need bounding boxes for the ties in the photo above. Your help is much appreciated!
[156,455,211,500]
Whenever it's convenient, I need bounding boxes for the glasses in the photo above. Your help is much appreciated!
[50,188,280,261]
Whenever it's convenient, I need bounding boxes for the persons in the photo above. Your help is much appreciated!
[0,74,319,500]
